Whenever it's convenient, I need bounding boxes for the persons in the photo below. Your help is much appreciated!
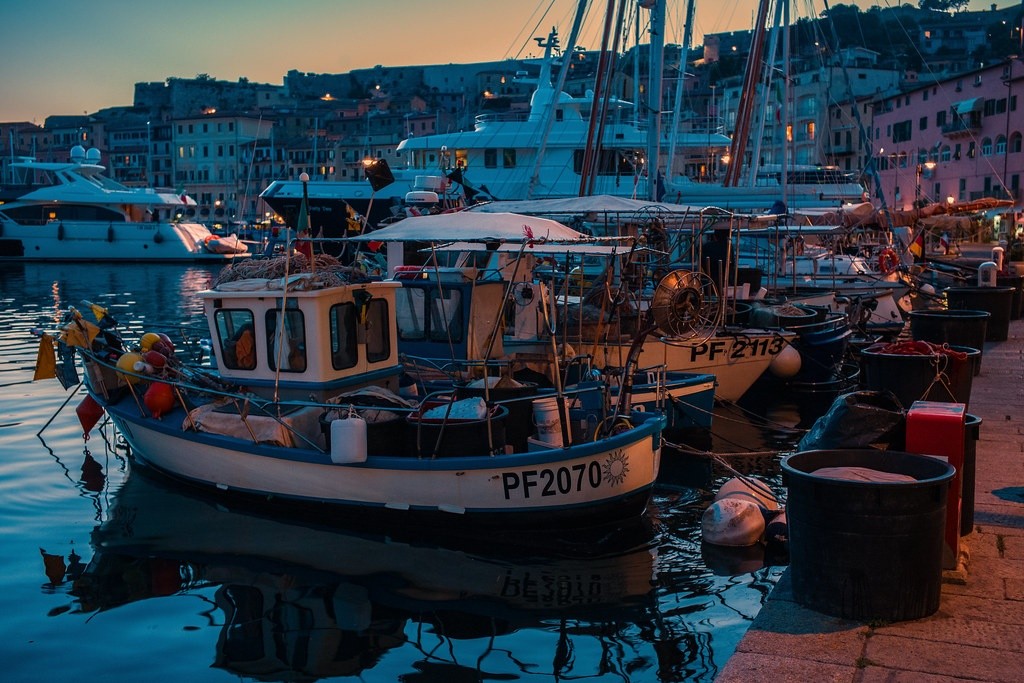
[153,208,159,222]
[143,211,152,222]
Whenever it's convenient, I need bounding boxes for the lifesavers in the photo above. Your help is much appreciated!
[205,234,220,243]
[879,248,899,273]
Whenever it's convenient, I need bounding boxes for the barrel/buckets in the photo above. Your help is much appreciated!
[965,274,1024,320]
[860,341,981,413]
[531,396,572,447]
[944,286,1016,342]
[331,418,367,463]
[907,309,991,377]
[896,411,983,536]
[723,297,860,431]
[780,447,958,625]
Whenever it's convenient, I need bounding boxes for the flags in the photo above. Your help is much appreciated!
[182,194,187,203]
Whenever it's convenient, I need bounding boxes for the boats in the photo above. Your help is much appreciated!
[31,0,1023,549]
[1,163,252,267]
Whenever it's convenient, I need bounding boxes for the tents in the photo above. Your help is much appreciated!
[413,196,850,336]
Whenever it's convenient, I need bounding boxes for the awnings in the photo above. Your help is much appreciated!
[298,213,628,384]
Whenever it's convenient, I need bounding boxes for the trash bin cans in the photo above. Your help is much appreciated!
[907,398,965,568]
